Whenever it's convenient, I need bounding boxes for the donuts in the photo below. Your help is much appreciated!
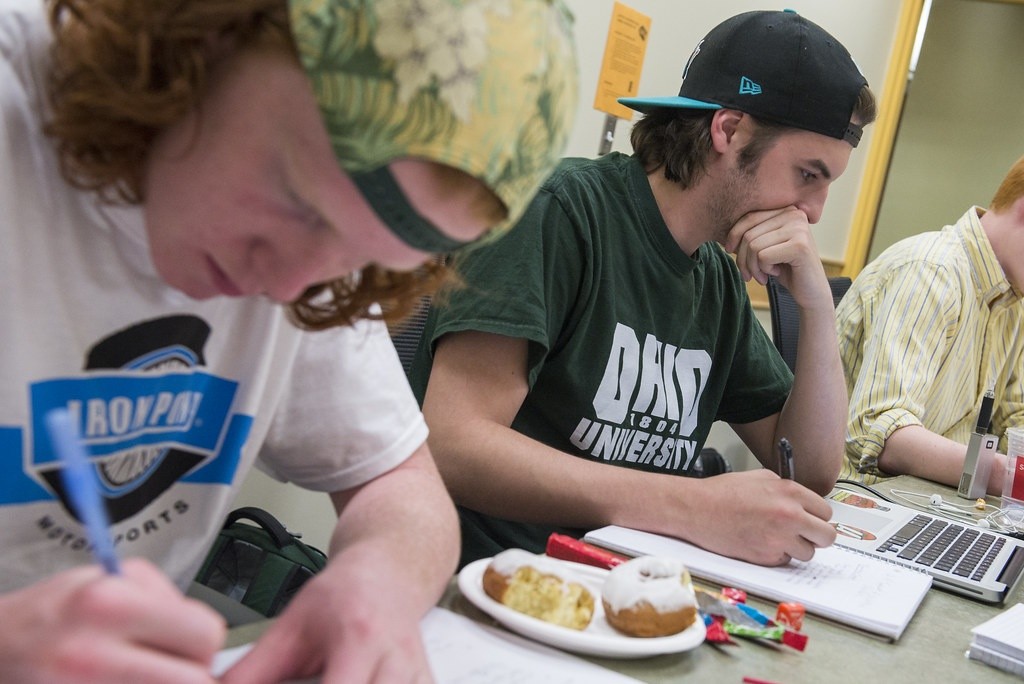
[601,554,697,638]
[483,548,595,631]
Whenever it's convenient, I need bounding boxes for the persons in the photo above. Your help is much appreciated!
[825,148,1021,490]
[408,7,879,578]
[2,1,586,684]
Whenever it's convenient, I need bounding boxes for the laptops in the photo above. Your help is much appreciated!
[817,487,1024,605]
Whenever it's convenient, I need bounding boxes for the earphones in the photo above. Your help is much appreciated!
[960,519,990,529]
[930,494,958,512]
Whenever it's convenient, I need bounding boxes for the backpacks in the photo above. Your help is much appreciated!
[194,506,327,619]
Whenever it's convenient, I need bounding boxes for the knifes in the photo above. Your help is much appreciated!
[546,532,782,650]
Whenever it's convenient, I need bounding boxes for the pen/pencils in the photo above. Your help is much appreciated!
[46,410,118,577]
[777,436,795,481]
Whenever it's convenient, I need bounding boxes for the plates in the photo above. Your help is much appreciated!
[456,556,708,659]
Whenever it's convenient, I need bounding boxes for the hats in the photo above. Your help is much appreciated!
[616,9,867,149]
[290,0,578,256]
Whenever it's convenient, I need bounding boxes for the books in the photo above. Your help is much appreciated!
[960,601,1024,679]
[582,516,945,647]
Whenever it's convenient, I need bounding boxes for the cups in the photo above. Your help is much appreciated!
[1000,427,1024,533]
